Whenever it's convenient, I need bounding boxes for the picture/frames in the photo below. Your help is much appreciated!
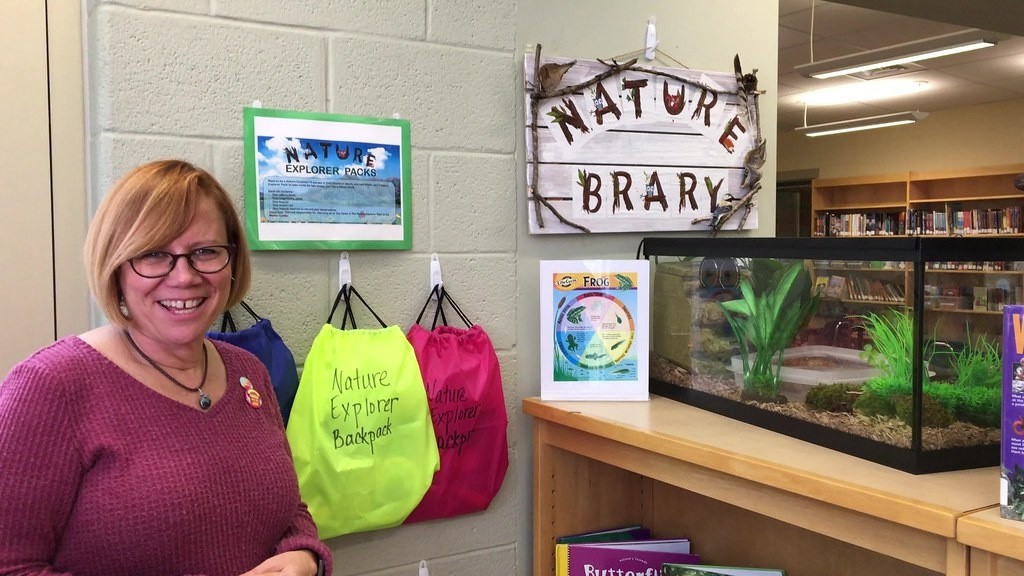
[239,106,417,254]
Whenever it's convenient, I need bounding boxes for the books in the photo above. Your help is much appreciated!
[556,522,786,576]
[810,202,1024,313]
[999,304,1024,520]
[538,259,655,406]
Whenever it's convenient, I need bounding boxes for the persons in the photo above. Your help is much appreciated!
[2,159,335,576]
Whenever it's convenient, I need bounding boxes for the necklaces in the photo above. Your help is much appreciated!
[119,324,213,412]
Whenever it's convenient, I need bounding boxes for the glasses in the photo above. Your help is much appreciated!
[121,242,240,280]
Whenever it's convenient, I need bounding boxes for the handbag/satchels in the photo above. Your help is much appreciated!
[404,283,510,527]
[284,284,440,540]
[198,295,300,431]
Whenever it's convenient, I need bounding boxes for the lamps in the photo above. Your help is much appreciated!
[796,0,995,86]
[792,96,930,143]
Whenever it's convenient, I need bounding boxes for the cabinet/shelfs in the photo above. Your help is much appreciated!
[805,176,1024,354]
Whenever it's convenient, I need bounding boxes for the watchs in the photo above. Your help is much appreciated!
[305,548,325,576]
